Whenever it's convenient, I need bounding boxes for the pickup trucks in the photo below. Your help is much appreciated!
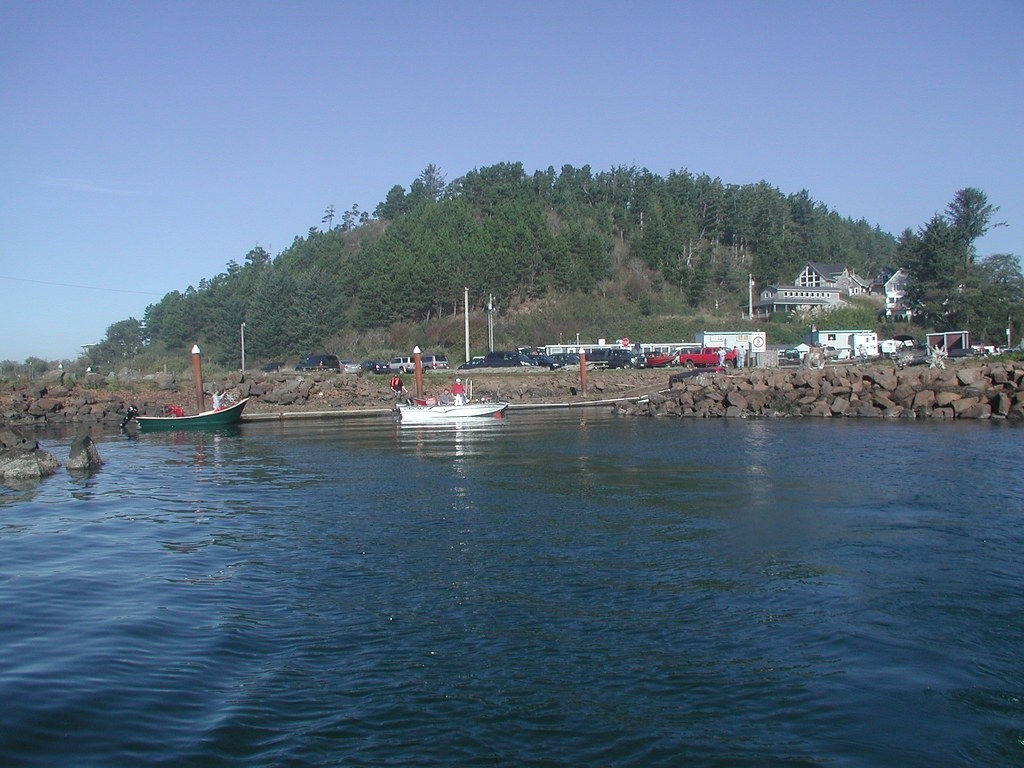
[679,347,736,368]
[589,350,647,369]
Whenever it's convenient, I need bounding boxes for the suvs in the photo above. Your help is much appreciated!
[386,356,422,373]
[421,355,450,372]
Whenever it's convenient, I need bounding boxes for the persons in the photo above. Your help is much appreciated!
[439,388,454,406]
[390,373,408,405]
[450,378,466,405]
[211,390,226,410]
[732,346,746,369]
[856,344,868,366]
[716,347,727,367]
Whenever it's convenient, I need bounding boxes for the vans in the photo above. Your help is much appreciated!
[294,354,341,373]
[484,351,539,368]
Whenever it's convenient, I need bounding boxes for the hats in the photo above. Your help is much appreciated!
[394,374,398,377]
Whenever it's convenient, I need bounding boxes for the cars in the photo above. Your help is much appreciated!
[456,358,484,370]
[360,359,391,375]
[338,360,363,376]
[259,362,284,373]
[549,353,579,366]
[528,354,566,370]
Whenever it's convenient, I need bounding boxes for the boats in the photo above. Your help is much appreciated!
[118,344,252,431]
[392,347,510,424]
